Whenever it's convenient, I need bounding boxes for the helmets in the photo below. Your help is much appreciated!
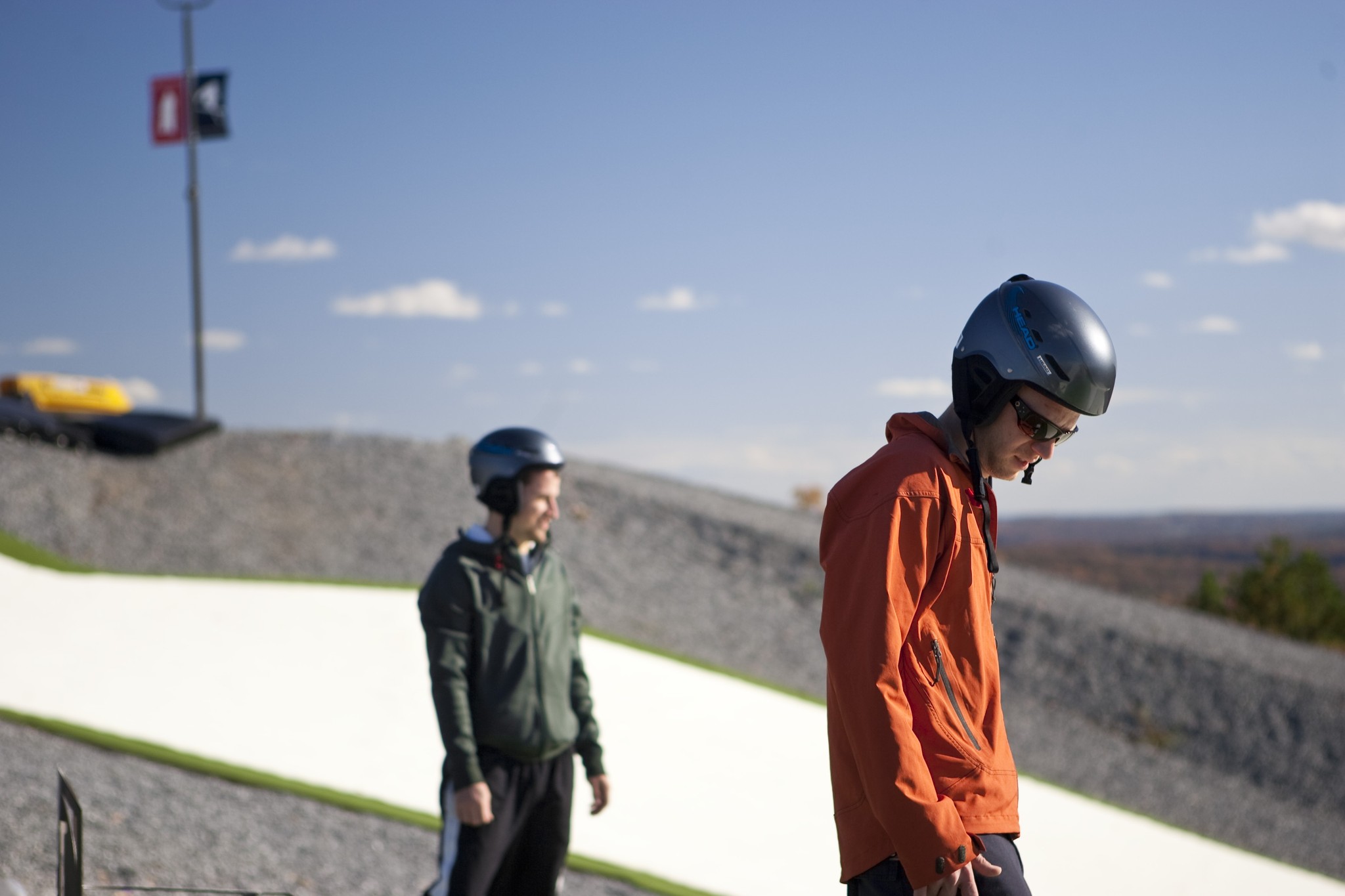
[468,427,564,490]
[953,273,1117,416]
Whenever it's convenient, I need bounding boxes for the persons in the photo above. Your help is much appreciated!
[818,274,1117,896]
[418,428,609,896]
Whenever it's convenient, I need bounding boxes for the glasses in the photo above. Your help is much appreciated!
[1010,393,1078,446]
